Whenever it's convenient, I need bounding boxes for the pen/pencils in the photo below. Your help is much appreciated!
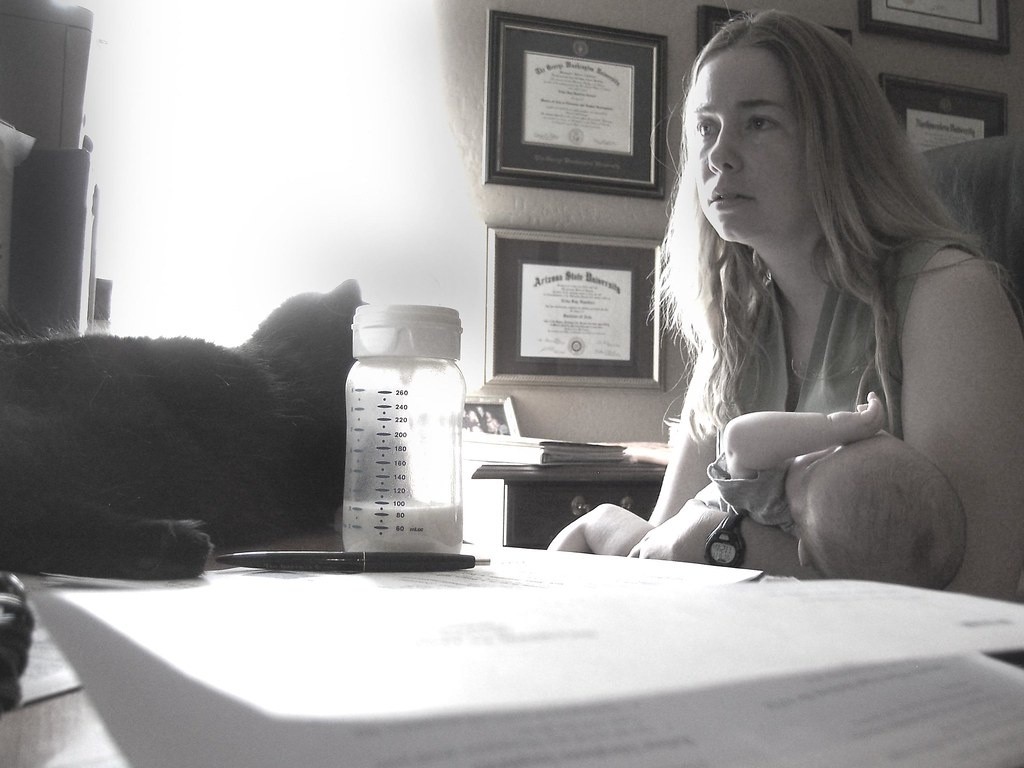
[213,551,492,575]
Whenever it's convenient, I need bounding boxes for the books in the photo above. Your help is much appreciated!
[460,431,630,467]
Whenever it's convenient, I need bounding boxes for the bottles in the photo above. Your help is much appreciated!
[341,304,465,554]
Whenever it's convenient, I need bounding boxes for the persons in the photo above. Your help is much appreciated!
[463,406,510,436]
[546,392,968,591]
[647,10,1024,605]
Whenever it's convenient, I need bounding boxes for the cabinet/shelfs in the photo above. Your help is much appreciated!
[457,462,670,553]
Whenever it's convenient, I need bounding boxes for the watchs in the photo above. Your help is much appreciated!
[704,509,749,569]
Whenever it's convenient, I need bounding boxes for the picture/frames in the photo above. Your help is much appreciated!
[484,225,667,390]
[481,8,666,198]
[859,0,1010,55]
[879,71,1007,153]
[462,394,521,438]
[697,5,853,76]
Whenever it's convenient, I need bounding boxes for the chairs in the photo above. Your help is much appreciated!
[913,133,1024,329]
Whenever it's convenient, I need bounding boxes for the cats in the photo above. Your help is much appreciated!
[1,278,372,583]
